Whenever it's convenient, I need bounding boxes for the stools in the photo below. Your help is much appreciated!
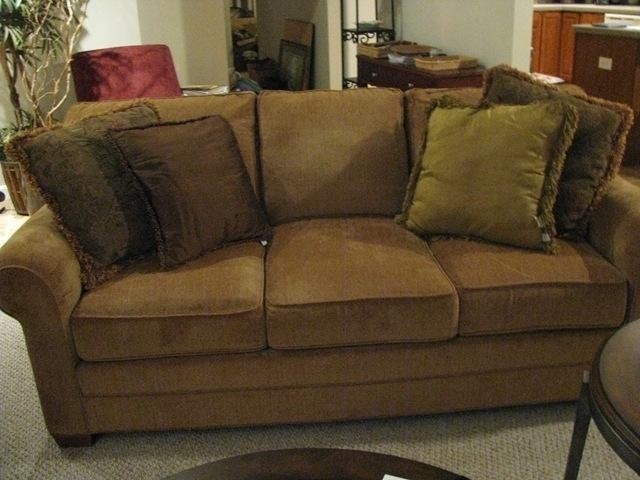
[564,321,640,478]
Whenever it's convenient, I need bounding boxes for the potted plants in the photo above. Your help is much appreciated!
[2,0,87,215]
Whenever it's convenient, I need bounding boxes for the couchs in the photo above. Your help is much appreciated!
[0,90,640,448]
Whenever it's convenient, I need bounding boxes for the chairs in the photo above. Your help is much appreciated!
[70,45,184,101]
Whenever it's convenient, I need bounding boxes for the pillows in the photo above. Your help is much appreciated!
[483,65,633,240]
[5,100,161,289]
[395,95,579,254]
[105,115,274,272]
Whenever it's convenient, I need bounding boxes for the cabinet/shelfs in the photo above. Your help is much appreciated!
[572,30,639,167]
[527,11,604,79]
